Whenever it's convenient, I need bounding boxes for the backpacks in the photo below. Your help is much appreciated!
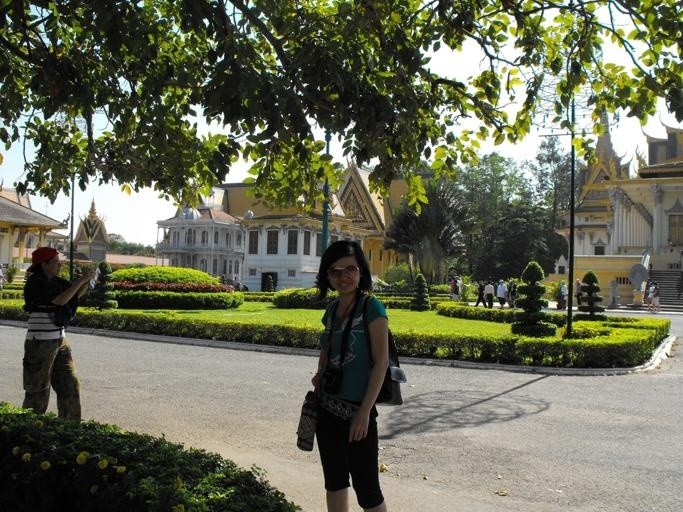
[363,294,406,406]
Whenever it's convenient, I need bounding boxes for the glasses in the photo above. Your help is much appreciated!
[326,265,359,279]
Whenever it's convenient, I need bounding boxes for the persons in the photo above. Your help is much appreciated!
[448,276,462,301]
[508,280,518,309]
[668,238,673,246]
[475,280,488,308]
[575,279,583,305]
[235,285,240,291]
[646,281,655,311]
[21,246,97,421]
[559,280,568,310]
[651,282,661,312]
[313,238,390,511]
[241,285,248,291]
[496,279,508,307]
[0,263,7,288]
[484,280,494,308]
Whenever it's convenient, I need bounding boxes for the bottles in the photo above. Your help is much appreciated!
[297,391,317,451]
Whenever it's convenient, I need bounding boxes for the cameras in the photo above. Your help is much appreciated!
[323,366,344,396]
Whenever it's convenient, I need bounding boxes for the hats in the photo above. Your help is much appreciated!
[26,247,59,273]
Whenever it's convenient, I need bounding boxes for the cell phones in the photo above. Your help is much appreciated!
[89,267,102,289]
